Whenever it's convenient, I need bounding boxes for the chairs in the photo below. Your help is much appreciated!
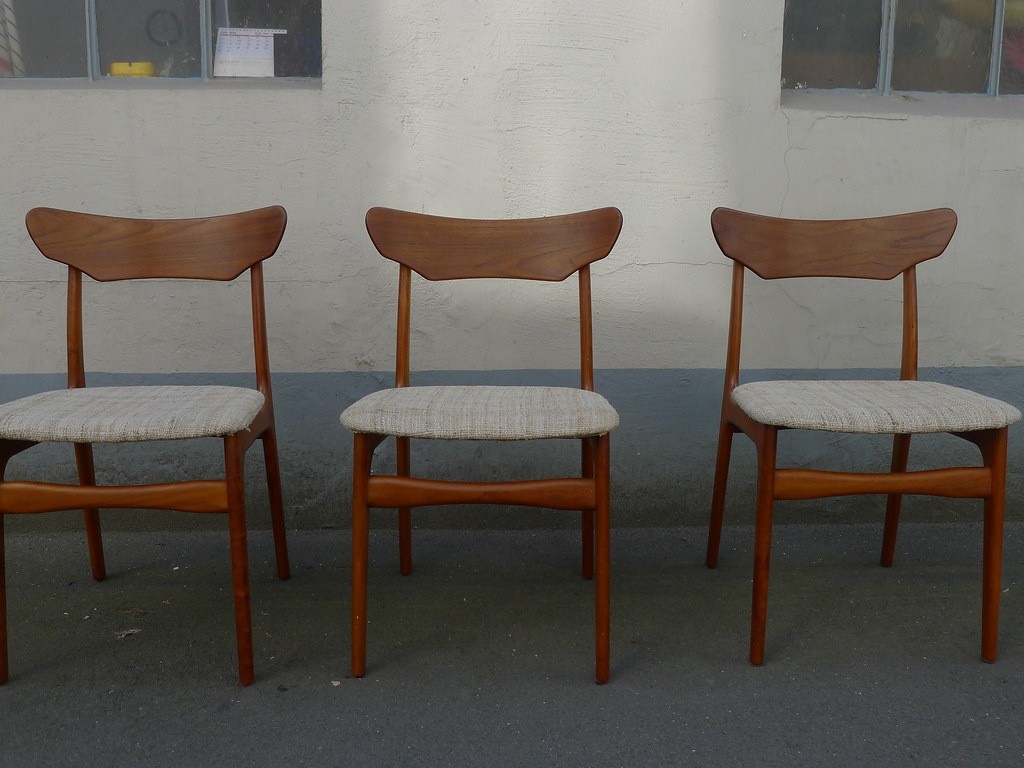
[707,204,1022,668]
[0,205,289,687]
[339,205,624,684]
